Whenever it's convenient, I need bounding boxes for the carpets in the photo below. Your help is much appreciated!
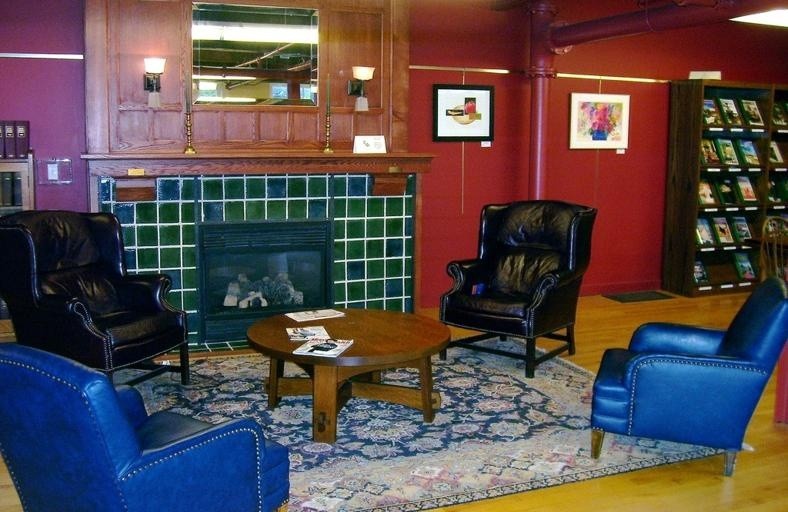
[601,290,674,303]
[114,338,755,512]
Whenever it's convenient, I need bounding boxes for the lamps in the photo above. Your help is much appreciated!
[191,21,319,103]
[348,66,375,111]
[143,57,166,107]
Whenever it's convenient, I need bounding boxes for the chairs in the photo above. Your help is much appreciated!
[439,199,597,377]
[0,343,290,512]
[591,278,788,476]
[757,215,787,282]
[0,211,190,388]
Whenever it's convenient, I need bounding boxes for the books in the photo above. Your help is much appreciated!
[0,120,30,158]
[285,307,347,322]
[0,172,21,208]
[285,326,330,342]
[293,338,354,358]
[691,96,788,290]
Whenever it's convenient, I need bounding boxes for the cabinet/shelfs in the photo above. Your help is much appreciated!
[665,78,788,297]
[0,150,38,344]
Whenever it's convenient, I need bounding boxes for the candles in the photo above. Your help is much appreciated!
[184,69,191,113]
[326,74,331,113]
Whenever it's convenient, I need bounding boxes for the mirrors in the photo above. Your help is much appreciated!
[179,0,329,113]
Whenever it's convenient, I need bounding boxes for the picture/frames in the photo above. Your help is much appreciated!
[570,91,631,150]
[432,83,495,141]
